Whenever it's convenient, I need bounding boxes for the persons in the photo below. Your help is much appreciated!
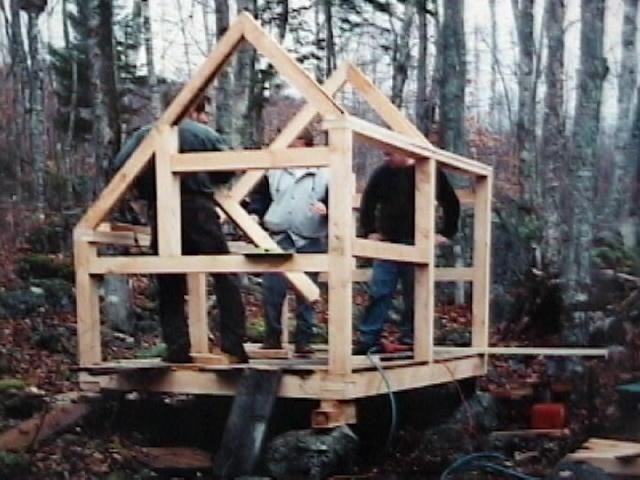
[352,148,460,355]
[112,94,248,363]
[241,128,328,354]
